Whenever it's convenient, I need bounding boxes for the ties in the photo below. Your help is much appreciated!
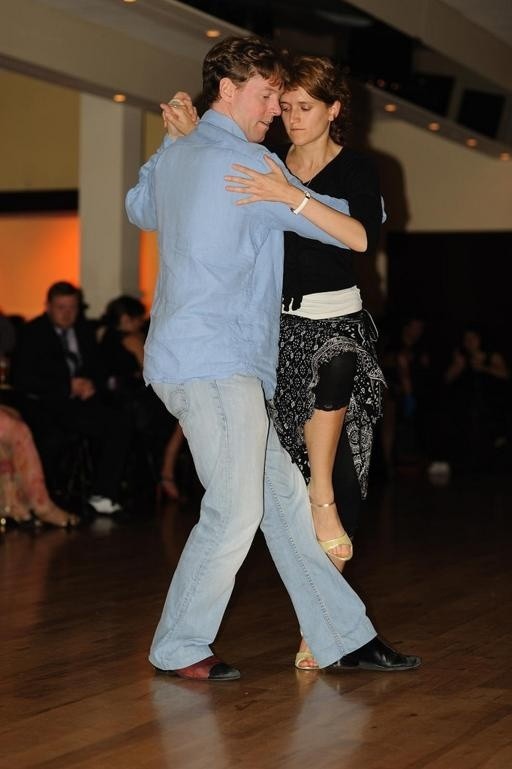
[61,329,68,351]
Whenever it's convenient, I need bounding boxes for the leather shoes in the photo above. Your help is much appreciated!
[329,637,422,672]
[154,655,241,682]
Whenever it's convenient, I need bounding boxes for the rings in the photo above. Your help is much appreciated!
[174,105,177,108]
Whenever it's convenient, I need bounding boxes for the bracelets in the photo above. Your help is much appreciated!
[290,190,311,215]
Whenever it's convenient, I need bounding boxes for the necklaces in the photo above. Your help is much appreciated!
[286,144,342,179]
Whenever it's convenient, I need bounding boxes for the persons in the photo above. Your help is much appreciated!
[153,674,393,768]
[125,33,424,684]
[0,527,77,635]
[160,52,391,671]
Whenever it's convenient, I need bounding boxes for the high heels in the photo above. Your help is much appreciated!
[85,497,140,522]
[155,482,191,506]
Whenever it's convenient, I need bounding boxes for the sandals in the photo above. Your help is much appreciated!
[1,503,81,527]
[307,482,353,561]
[294,628,320,670]
[2,529,80,551]
